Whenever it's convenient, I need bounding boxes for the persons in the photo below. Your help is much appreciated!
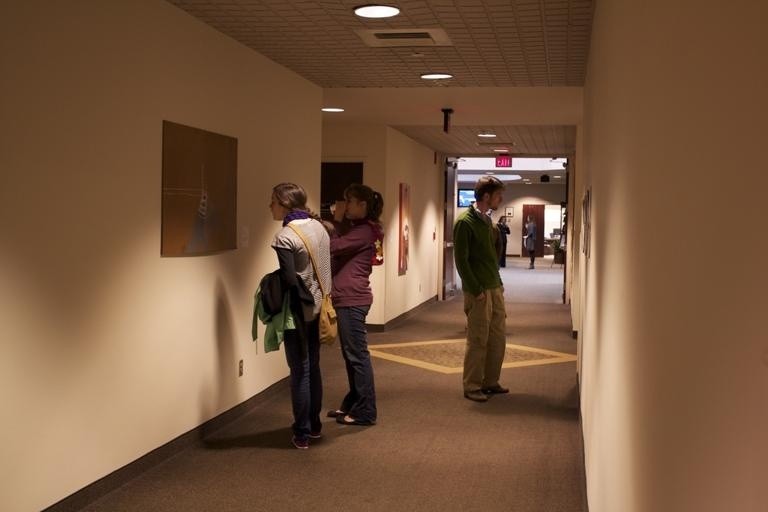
[522,214,537,270]
[326,183,384,428]
[496,215,510,267]
[452,175,511,402]
[269,181,338,450]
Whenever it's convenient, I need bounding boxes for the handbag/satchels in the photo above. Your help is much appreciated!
[319,294,338,346]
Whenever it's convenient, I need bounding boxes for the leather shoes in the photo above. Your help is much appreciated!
[464,389,489,401]
[327,407,376,424]
[481,385,510,394]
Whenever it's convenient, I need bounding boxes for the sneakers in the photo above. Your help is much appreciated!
[289,429,322,448]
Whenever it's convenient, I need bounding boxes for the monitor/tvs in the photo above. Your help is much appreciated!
[458,188,476,207]
